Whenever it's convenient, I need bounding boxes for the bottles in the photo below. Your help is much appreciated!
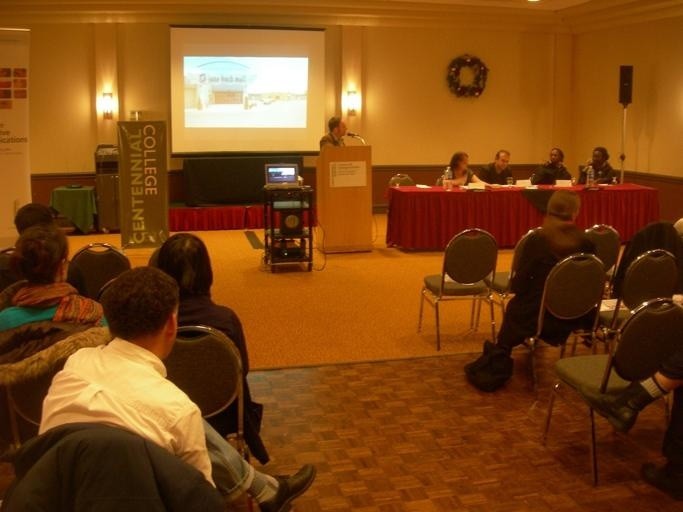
[586,165,595,187]
[571,175,576,186]
[507,178,514,187]
[444,166,453,192]
[611,176,617,186]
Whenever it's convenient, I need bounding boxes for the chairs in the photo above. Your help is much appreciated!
[389,173,414,186]
[436,178,443,186]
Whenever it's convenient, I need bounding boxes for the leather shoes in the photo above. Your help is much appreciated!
[640,459,683,500]
[258,463,317,511]
[578,379,656,435]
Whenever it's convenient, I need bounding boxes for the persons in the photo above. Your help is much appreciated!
[14,202,54,236]
[442,150,492,191]
[578,341,683,502]
[154,232,270,466]
[478,149,512,186]
[0,223,110,332]
[577,147,619,184]
[464,189,597,392]
[36,264,316,512]
[319,116,346,149]
[530,147,572,185]
[197,73,213,112]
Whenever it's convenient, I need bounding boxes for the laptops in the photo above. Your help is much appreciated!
[265,163,301,191]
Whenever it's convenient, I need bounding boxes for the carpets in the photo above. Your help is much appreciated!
[57,213,626,371]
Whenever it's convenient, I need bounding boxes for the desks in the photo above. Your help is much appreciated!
[50,186,95,234]
[385,182,661,253]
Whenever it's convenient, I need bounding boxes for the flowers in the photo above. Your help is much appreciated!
[447,54,490,98]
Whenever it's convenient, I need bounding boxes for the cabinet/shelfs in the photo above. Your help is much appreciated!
[94,144,121,232]
[262,184,314,273]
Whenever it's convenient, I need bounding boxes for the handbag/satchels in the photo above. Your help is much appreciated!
[463,338,516,393]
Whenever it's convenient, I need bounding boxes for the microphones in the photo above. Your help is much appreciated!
[347,132,358,137]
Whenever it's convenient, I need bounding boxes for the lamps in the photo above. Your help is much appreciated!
[346,89,360,118]
[101,92,114,120]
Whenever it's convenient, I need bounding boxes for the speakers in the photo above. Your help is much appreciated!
[618,65,634,103]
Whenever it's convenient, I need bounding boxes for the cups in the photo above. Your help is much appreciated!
[395,177,400,189]
